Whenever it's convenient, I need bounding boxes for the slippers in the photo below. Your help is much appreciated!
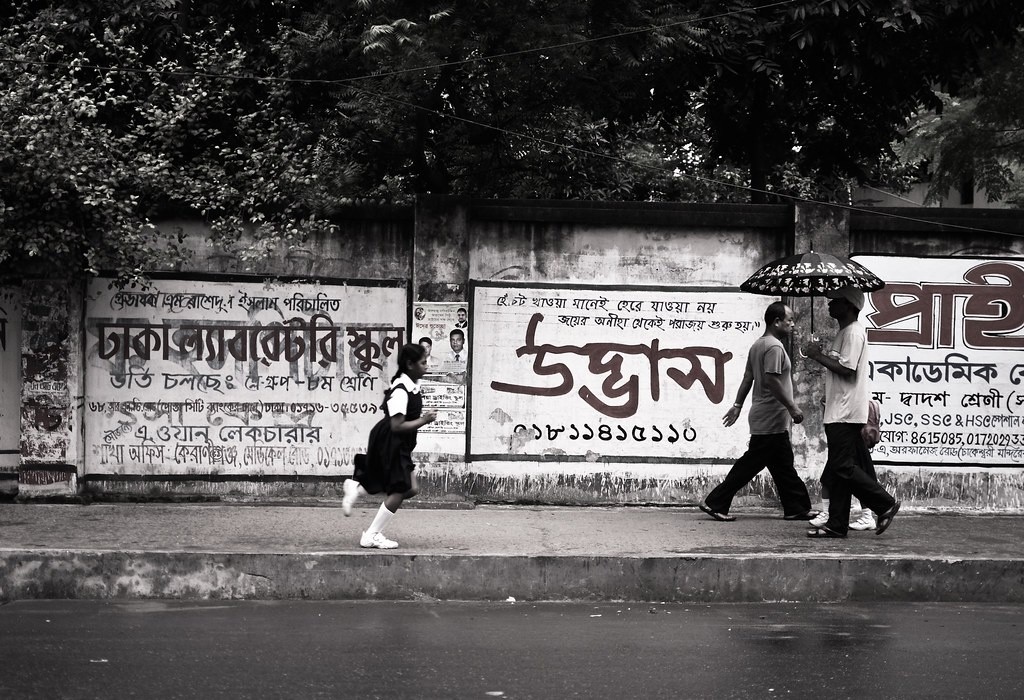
[876,500,901,535]
[806,527,848,538]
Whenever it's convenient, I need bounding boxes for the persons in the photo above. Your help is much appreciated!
[444,329,468,362]
[342,342,438,549]
[419,337,438,364]
[455,308,468,328]
[808,396,877,531]
[806,285,900,538]
[699,302,822,520]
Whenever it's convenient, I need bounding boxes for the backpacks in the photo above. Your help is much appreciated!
[861,398,880,449]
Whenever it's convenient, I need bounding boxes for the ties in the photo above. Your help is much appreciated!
[455,354,460,361]
[459,325,461,328]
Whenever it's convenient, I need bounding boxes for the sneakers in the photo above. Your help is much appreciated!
[342,478,360,517]
[849,514,876,530]
[808,508,830,526]
[360,530,399,548]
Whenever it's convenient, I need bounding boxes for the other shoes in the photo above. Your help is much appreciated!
[699,503,737,521]
[783,510,822,520]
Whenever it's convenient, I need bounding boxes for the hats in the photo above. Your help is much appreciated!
[824,284,865,310]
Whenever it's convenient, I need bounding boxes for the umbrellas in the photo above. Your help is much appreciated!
[740,251,885,358]
